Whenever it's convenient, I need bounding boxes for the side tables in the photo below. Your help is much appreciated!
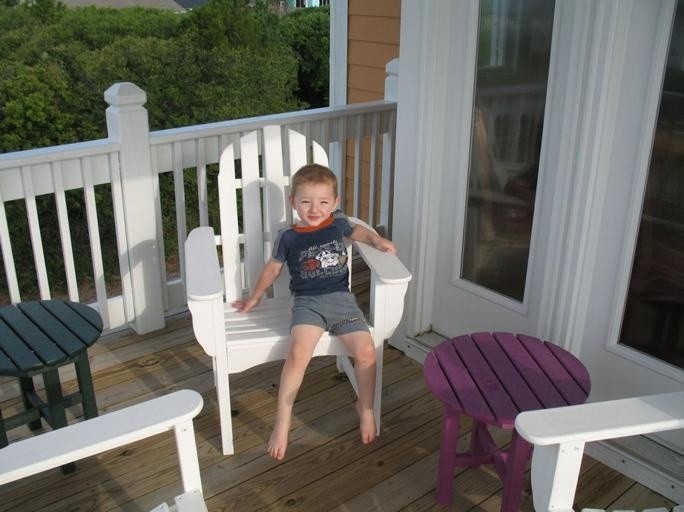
[0,301,104,474]
[422,331,591,512]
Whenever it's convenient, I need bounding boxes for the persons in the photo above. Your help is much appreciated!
[231,164,397,461]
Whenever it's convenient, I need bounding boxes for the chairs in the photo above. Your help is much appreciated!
[183,123,413,457]
[0,389,210,512]
[467,95,532,280]
[514,389,684,512]
